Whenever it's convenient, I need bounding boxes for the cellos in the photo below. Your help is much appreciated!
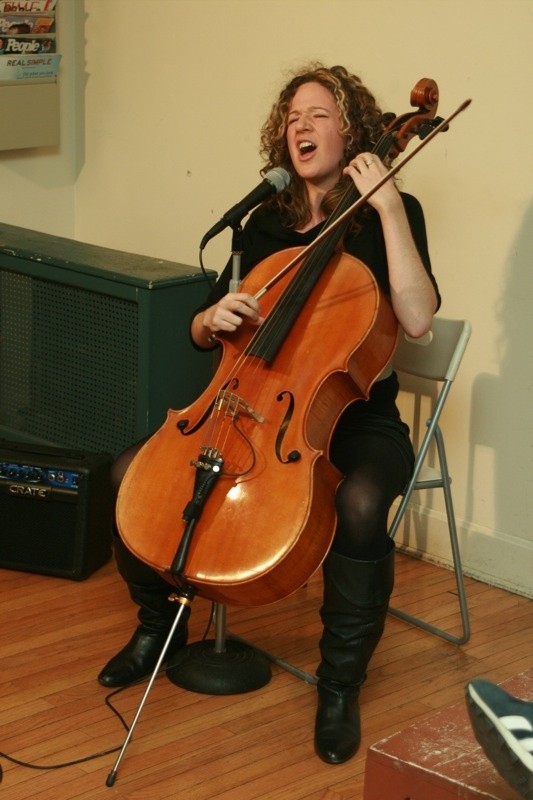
[105,77,441,788]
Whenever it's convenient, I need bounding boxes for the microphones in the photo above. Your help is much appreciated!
[201,166,292,253]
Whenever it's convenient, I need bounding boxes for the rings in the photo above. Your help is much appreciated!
[366,159,376,166]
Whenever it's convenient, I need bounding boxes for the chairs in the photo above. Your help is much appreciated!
[211,316,472,685]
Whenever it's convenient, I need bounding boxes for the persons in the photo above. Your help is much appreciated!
[464,678,533,800]
[98,67,442,765]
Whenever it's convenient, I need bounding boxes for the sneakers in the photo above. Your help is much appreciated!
[464,676,533,800]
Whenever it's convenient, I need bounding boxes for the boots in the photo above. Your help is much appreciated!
[313,537,396,765]
[97,521,191,688]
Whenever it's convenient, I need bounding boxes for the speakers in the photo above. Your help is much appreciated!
[1,436,113,583]
